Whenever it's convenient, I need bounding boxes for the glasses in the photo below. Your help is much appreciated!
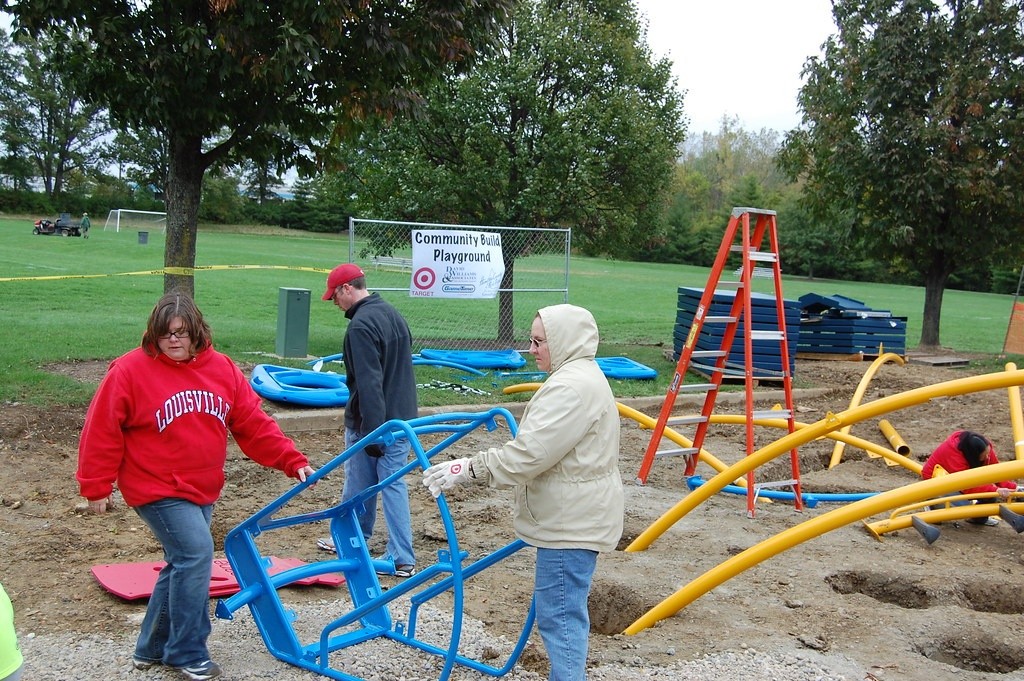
[528,337,547,347]
[157,329,190,339]
[330,283,353,301]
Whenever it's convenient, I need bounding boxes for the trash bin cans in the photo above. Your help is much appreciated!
[138,231,149,245]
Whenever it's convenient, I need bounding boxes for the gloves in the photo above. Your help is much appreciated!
[422,457,469,498]
[364,444,385,459]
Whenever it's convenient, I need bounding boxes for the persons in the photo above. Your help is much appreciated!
[317,265,419,579]
[75,292,321,681]
[425,302,624,681]
[81,213,91,239]
[920,430,1024,527]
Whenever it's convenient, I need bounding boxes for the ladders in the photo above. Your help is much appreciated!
[633,204,807,520]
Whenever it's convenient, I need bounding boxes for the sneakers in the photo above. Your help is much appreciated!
[132,656,164,670]
[375,564,415,577]
[182,658,223,680]
[317,536,337,551]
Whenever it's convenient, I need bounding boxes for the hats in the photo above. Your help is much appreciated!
[322,264,364,300]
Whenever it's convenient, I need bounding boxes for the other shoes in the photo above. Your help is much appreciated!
[965,516,999,526]
